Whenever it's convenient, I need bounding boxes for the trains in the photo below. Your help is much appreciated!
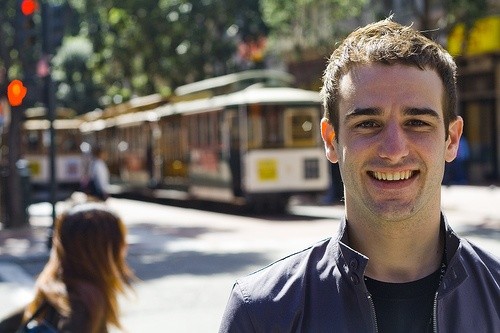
[74,69,333,216]
[1,107,86,199]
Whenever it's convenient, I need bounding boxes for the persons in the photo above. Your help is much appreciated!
[87,145,112,203]
[219,19,500,333]
[14,201,134,333]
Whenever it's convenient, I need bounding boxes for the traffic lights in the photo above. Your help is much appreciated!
[20,0,37,17]
[6,79,28,107]
